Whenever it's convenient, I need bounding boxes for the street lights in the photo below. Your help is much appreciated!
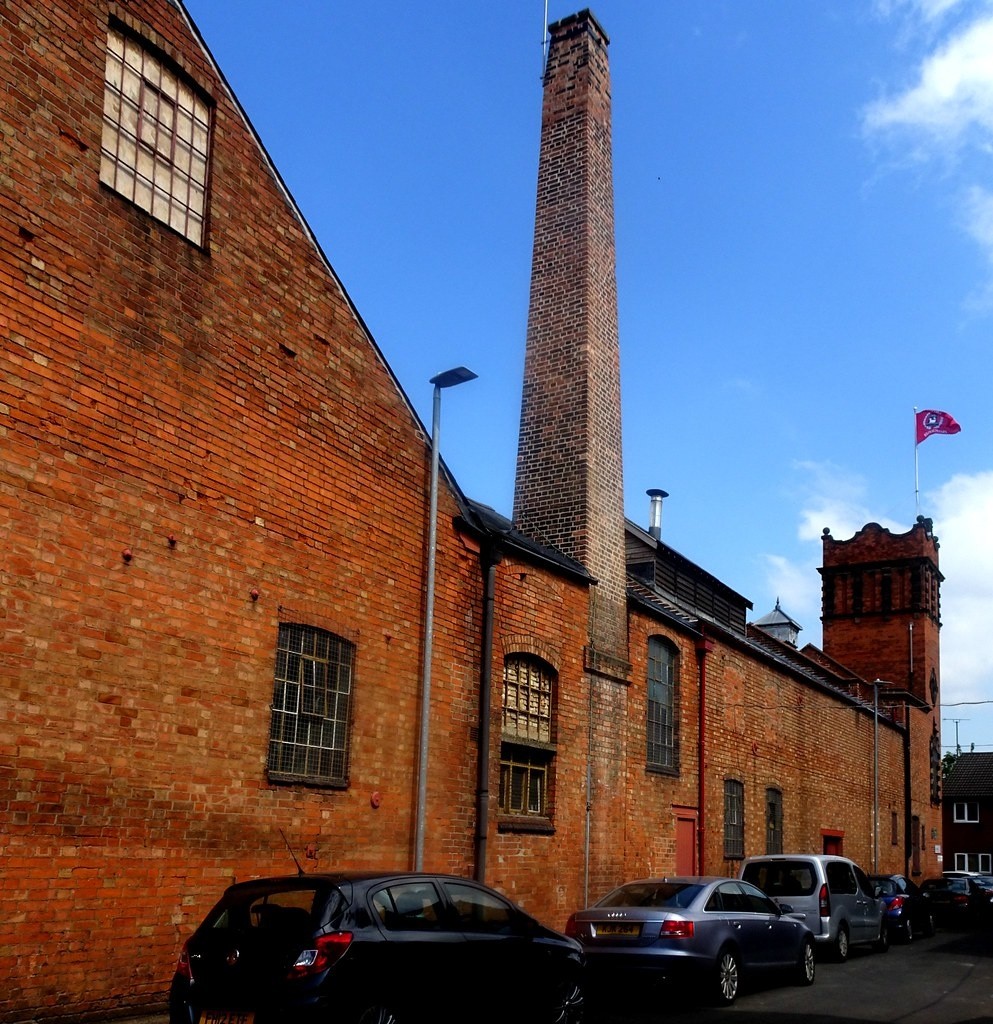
[413,367,478,870]
[874,679,894,876]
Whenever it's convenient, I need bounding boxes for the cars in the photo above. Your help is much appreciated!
[943,871,993,913]
[169,870,590,1024]
[918,878,983,928]
[565,874,817,1005]
[867,873,934,946]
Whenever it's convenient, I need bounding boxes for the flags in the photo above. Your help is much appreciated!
[916,409,962,444]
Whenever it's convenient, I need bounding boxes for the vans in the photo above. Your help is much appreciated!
[740,853,885,963]
